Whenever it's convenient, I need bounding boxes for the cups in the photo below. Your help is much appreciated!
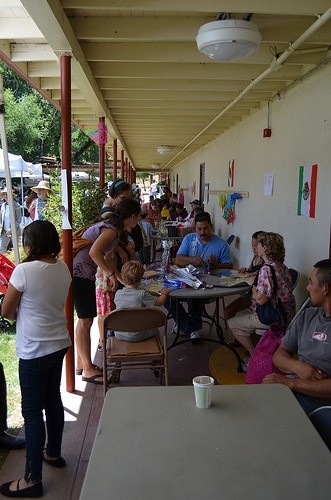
[193,376,214,408]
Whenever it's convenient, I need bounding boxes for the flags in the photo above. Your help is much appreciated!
[227,207,235,224]
[222,208,231,224]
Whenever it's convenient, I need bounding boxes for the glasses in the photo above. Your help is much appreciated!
[118,195,127,201]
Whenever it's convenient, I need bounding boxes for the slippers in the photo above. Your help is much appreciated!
[76,364,103,375]
[82,374,112,385]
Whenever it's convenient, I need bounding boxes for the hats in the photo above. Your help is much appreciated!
[189,200,199,204]
[30,180,55,196]
[0,186,17,200]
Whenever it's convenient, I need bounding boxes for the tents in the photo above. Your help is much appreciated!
[0,149,100,227]
[59,171,98,184]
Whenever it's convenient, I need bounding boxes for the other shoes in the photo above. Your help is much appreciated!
[175,348,188,361]
[237,356,252,373]
[0,478,44,499]
[42,448,66,467]
[190,330,201,343]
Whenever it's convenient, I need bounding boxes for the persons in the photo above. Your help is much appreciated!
[163,211,234,340]
[0,218,72,500]
[261,258,331,414]
[131,179,205,259]
[222,231,266,354]
[226,231,294,373]
[107,259,170,385]
[0,359,26,451]
[0,179,55,254]
[94,177,137,353]
[72,195,143,384]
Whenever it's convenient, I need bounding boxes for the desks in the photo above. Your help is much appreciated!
[149,236,186,265]
[137,267,258,374]
[165,226,193,237]
[79,383,331,500]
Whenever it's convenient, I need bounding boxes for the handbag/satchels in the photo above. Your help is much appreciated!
[56,226,91,262]
[255,265,286,325]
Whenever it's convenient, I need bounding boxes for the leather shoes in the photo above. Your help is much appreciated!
[0,432,26,450]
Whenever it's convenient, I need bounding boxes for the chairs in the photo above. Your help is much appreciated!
[255,296,312,336]
[286,269,300,295]
[165,296,228,337]
[100,307,169,398]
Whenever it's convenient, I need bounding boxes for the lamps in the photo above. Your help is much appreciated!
[157,145,173,155]
[195,19,264,63]
[152,163,160,170]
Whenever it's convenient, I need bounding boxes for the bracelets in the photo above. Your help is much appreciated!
[108,271,115,277]
[107,271,115,277]
[160,292,167,296]
